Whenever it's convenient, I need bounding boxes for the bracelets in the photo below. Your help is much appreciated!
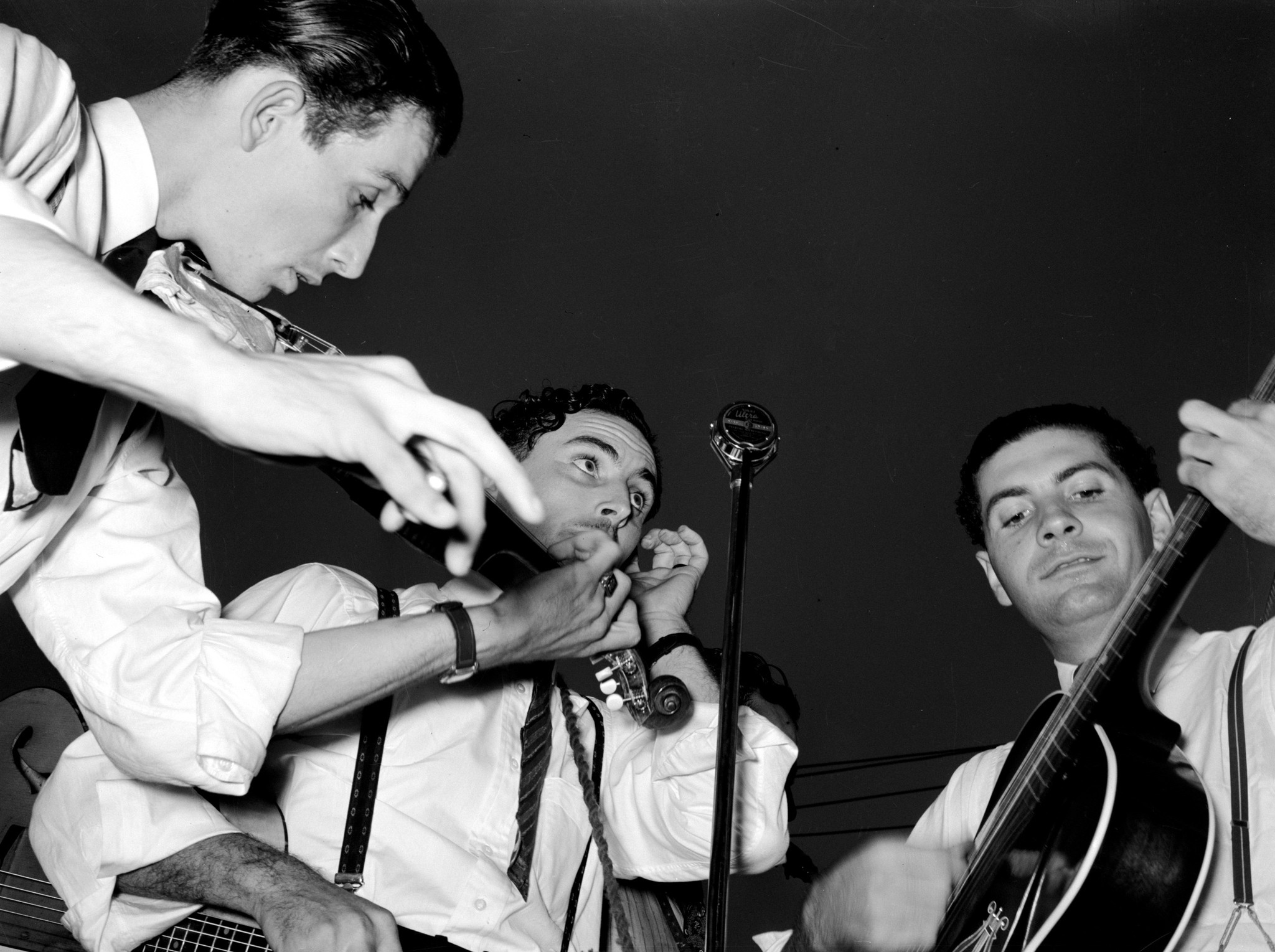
[646,632,705,671]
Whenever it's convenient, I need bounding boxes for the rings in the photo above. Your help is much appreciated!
[600,571,618,597]
[672,564,688,568]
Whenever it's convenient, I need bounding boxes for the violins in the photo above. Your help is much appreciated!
[141,239,693,734]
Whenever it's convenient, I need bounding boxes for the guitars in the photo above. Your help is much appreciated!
[0,677,474,951]
[932,355,1275,952]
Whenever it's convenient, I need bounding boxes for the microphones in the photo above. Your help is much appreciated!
[707,400,785,468]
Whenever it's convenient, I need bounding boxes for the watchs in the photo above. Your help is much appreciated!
[426,600,479,685]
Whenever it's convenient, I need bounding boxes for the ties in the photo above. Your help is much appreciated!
[506,664,559,901]
[3,225,177,511]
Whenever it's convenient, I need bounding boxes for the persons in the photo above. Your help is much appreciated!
[805,397,1274,952]
[0,0,822,952]
